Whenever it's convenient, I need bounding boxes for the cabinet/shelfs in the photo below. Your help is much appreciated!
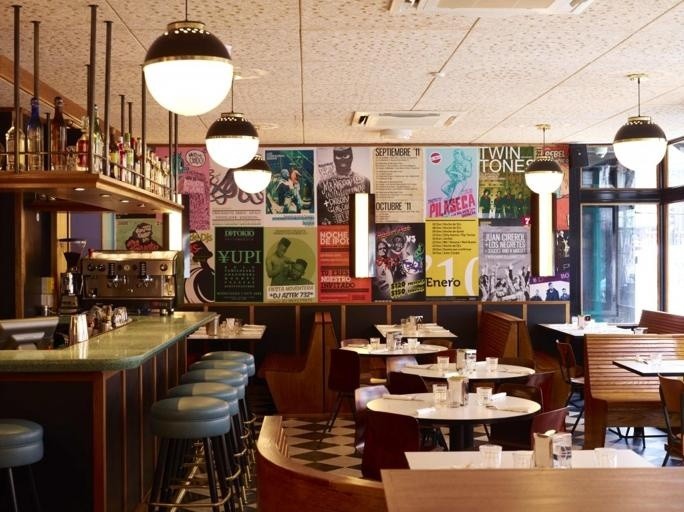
[0,4,185,214]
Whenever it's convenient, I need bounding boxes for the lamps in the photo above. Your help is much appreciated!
[524,124,565,195]
[204,72,260,169]
[141,0,235,117]
[234,124,273,194]
[613,73,668,172]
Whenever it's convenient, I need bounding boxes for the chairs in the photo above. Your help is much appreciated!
[341,339,381,381]
[657,373,684,467]
[361,411,444,482]
[531,406,574,451]
[319,348,385,444]
[188,360,256,465]
[555,339,622,441]
[422,339,452,349]
[386,356,419,382]
[388,371,429,395]
[487,384,543,452]
[432,349,467,364]
[354,386,391,456]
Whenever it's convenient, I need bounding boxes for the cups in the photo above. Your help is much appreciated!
[370,338,380,352]
[433,384,447,408]
[512,451,535,468]
[438,356,450,371]
[595,448,618,468]
[485,357,499,371]
[577,314,591,330]
[447,377,463,408]
[385,315,423,352]
[227,318,235,329]
[552,432,573,469]
[479,444,501,468]
[651,353,662,367]
[476,386,492,405]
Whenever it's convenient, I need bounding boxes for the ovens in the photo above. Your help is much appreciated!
[79,249,184,315]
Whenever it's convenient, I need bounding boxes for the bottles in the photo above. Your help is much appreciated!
[145,151,170,200]
[117,136,126,181]
[26,99,43,170]
[6,97,25,173]
[51,96,67,171]
[108,126,118,179]
[96,111,104,172]
[121,133,134,184]
[76,116,95,172]
[135,137,142,187]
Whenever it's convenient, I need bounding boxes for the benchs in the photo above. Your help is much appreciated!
[423,311,518,362]
[258,310,324,414]
[490,309,575,414]
[635,309,684,334]
[323,311,384,414]
[582,334,684,451]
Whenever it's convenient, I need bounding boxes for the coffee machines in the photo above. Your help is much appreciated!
[57,238,87,314]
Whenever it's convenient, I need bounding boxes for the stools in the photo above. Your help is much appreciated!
[1,417,44,512]
[166,383,248,506]
[201,351,257,450]
[181,369,254,482]
[148,396,231,512]
